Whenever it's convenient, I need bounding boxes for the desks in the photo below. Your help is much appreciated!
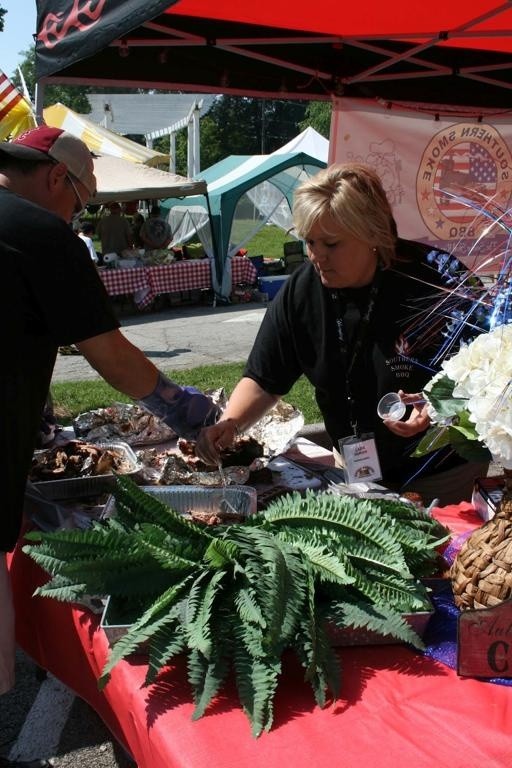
[6,437,512,768]
[96,255,258,309]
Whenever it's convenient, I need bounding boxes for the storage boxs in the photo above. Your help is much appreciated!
[258,275,291,300]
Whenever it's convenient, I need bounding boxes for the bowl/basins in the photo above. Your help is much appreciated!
[118,260,136,268]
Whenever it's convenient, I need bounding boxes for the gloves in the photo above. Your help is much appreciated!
[130,372,223,441]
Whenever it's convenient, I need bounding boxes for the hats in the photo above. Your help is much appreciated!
[0,128,97,197]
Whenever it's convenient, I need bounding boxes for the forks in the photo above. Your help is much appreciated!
[217,458,238,514]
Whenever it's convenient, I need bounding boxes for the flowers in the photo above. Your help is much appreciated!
[443,323,512,471]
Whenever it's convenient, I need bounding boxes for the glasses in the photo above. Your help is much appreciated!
[65,171,85,221]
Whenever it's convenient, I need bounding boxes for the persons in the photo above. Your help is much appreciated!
[138,204,174,251]
[94,202,133,254]
[121,198,146,250]
[58,220,109,355]
[0,127,218,708]
[191,157,511,511]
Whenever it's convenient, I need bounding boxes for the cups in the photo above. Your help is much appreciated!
[377,392,406,421]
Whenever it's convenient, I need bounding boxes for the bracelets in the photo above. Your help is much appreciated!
[128,246,132,249]
[222,418,241,438]
